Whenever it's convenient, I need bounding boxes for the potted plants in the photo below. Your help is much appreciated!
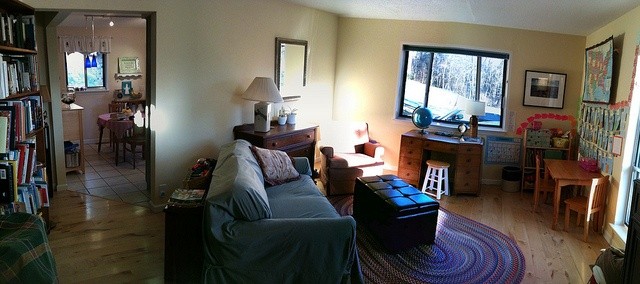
[287,106,298,125]
[278,106,287,125]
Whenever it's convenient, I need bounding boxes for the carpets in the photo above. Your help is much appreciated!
[327,194,526,283]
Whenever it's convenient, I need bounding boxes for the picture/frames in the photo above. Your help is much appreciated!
[523,70,568,109]
[582,35,613,104]
[122,81,132,96]
[274,36,308,103]
[118,57,137,74]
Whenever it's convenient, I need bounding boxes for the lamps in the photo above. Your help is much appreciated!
[241,77,283,133]
[84,15,91,69]
[464,100,485,138]
[91,16,98,66]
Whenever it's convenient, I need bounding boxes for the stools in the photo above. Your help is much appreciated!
[421,160,451,200]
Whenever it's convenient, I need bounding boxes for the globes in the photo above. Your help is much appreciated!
[412,105,433,134]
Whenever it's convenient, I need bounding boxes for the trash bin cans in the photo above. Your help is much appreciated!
[502,165,522,192]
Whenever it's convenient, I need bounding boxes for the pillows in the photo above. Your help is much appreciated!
[249,144,300,186]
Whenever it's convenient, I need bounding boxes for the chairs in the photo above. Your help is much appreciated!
[533,154,554,214]
[123,116,146,169]
[564,175,609,242]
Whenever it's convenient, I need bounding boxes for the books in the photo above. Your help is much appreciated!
[0,13,36,50]
[0,162,50,215]
[0,95,43,155]
[0,134,38,205]
[0,52,39,101]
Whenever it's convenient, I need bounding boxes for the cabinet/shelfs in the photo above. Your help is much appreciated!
[62,103,87,174]
[0,0,50,236]
[233,125,319,174]
[521,132,574,193]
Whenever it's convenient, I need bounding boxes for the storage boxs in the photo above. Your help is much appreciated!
[526,128,551,147]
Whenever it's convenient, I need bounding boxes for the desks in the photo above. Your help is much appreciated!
[396,129,485,196]
[111,96,146,115]
[543,158,609,231]
[98,112,135,167]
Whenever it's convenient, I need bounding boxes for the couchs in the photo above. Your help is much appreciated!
[202,139,365,283]
[316,119,385,196]
[354,173,440,253]
